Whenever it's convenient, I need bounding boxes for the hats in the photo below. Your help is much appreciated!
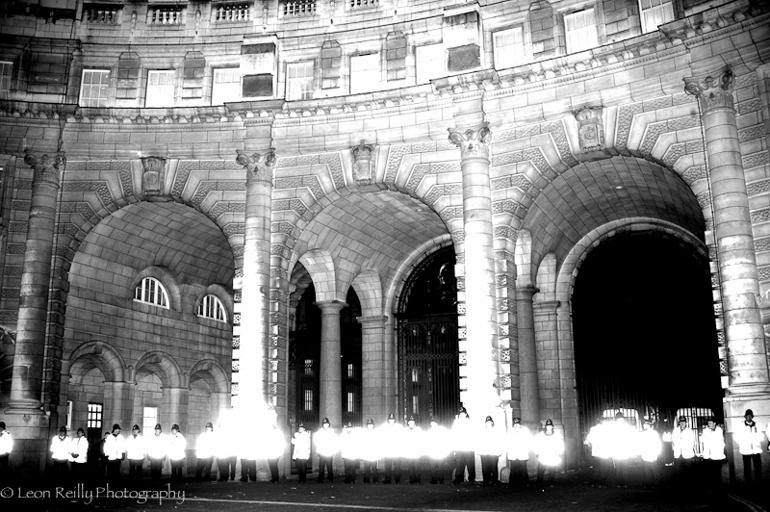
[58,420,179,433]
[595,412,719,425]
[744,409,754,419]
[0,421,6,428]
[298,406,555,428]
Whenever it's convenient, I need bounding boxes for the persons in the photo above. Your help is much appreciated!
[582,409,770,489]
[0,420,13,486]
